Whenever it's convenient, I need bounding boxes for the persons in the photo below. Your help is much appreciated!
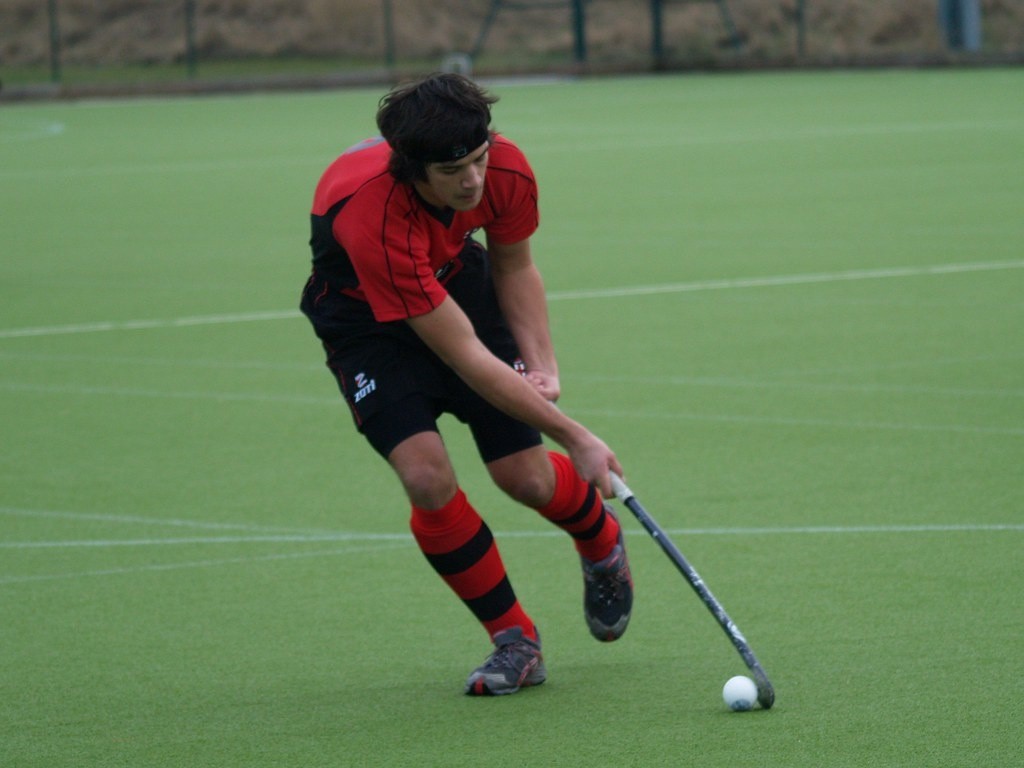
[296,69,635,695]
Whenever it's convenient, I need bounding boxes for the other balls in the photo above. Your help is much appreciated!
[722,675,757,711]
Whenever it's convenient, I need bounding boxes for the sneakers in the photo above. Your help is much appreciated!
[581,504,633,645]
[465,625,547,697]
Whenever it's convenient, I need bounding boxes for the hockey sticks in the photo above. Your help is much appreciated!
[608,467,778,711]
